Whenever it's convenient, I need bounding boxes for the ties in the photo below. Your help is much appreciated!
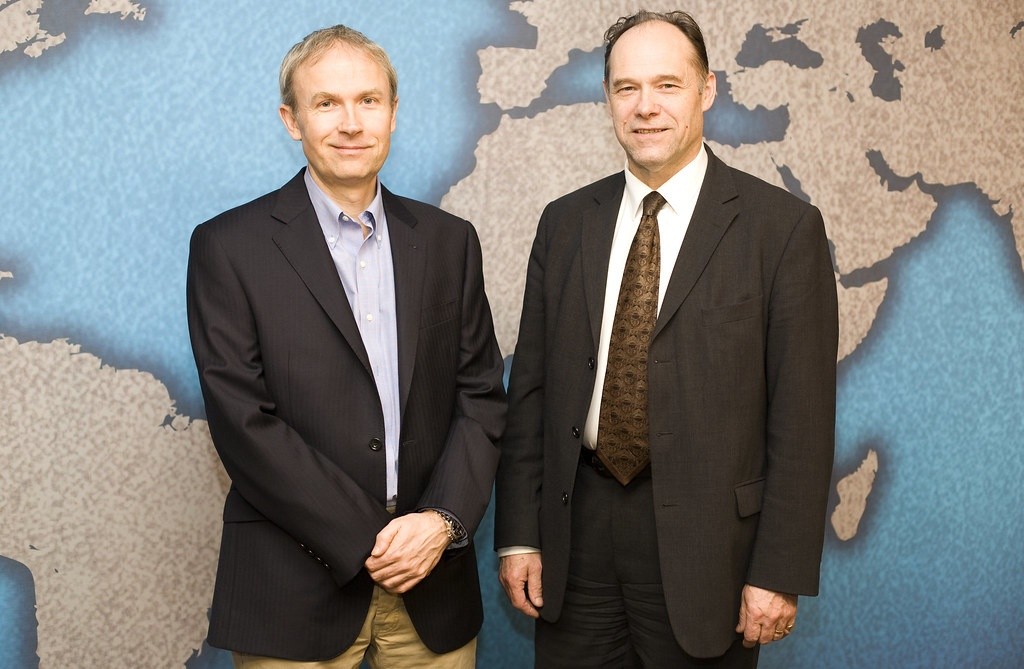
[596,191,667,486]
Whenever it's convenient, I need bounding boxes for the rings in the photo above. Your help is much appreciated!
[787,624,794,629]
[775,629,784,634]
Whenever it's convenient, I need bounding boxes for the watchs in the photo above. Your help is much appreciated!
[432,508,466,544]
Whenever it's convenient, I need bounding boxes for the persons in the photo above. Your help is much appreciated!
[185,20,510,669]
[487,10,840,668]
